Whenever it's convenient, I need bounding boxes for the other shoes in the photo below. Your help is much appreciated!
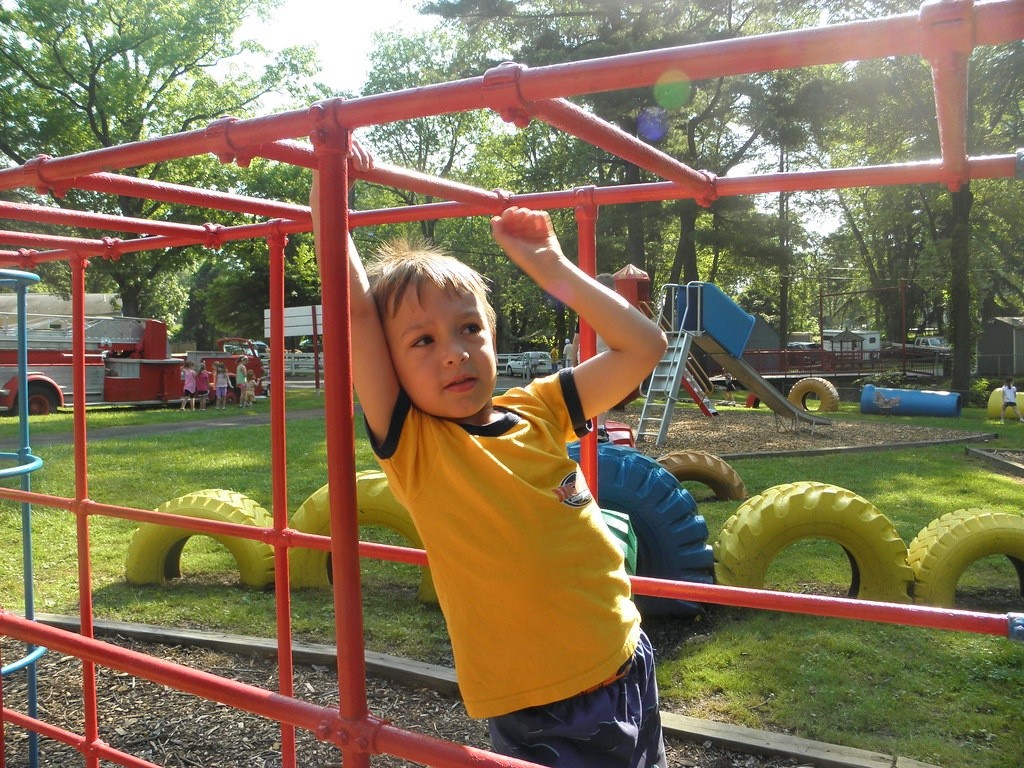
[223,407,226,411]
[1001,418,1005,421]
[216,408,220,411]
[1020,418,1024,422]
[180,408,185,411]
[239,405,243,409]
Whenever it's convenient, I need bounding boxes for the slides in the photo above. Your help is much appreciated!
[692,329,830,424]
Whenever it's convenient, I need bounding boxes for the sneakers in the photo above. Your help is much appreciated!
[728,401,735,406]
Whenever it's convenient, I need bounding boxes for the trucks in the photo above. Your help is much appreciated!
[892,337,953,363]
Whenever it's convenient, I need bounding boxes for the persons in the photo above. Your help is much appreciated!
[180,360,234,411]
[744,392,760,408]
[284,347,292,380]
[1001,376,1024,422]
[261,372,270,394]
[309,138,668,768]
[102,350,115,375]
[717,368,736,405]
[563,339,573,368]
[572,273,615,443]
[235,355,260,410]
[263,343,270,370]
[548,342,558,375]
[290,346,302,376]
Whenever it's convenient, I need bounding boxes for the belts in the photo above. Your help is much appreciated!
[569,652,634,698]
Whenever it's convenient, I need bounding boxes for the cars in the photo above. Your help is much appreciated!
[224,341,270,357]
[786,341,820,367]
[506,352,560,377]
[261,369,270,397]
[300,339,319,346]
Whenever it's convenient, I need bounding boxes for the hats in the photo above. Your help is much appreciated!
[565,339,570,343]
